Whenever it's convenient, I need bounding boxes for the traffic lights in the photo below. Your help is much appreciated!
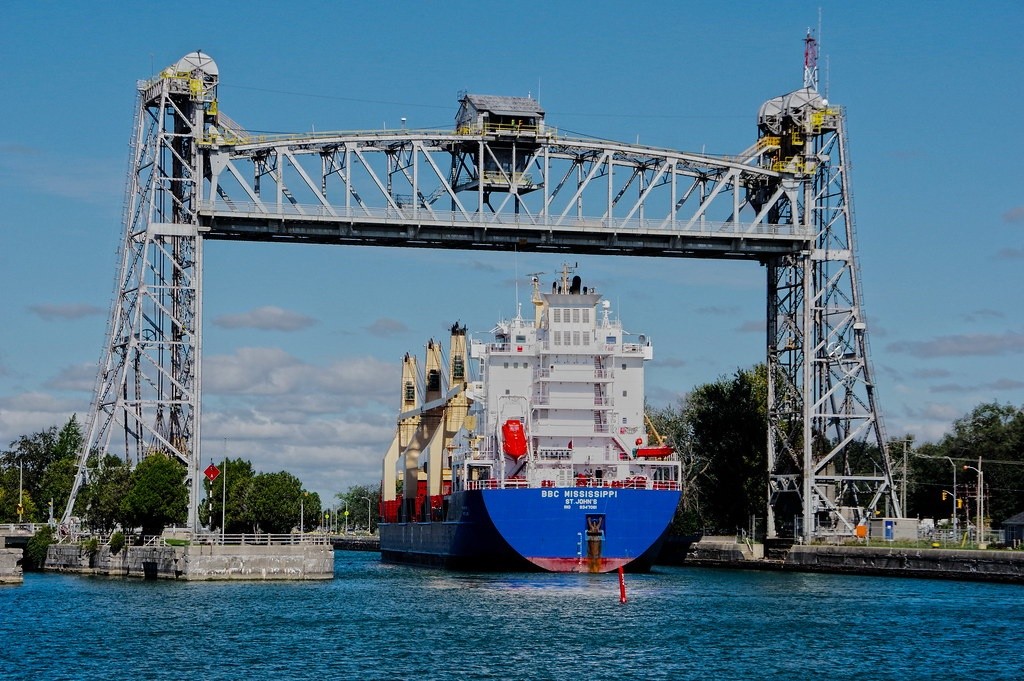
[943,493,947,499]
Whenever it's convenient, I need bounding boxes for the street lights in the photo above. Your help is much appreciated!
[964,466,985,543]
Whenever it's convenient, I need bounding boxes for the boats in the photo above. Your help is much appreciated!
[379,258,682,576]
